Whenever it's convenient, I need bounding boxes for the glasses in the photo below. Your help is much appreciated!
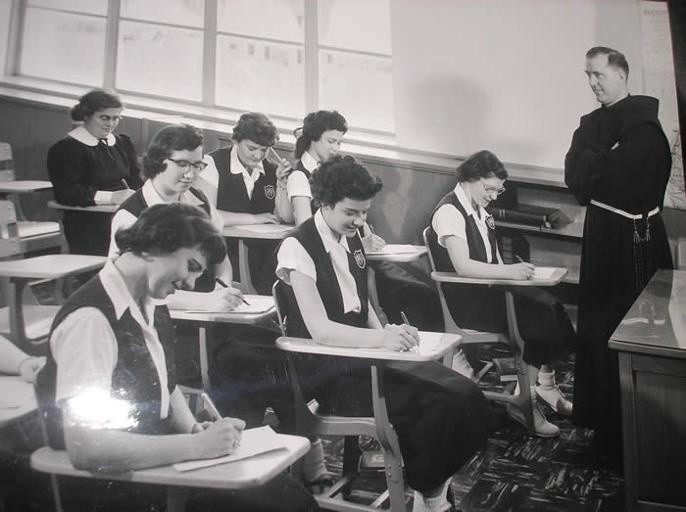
[168,157,208,173]
[481,179,506,196]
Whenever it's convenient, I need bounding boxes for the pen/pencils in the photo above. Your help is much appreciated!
[401,311,419,348]
[369,223,376,235]
[515,254,524,263]
[214,276,250,306]
[121,178,130,189]
[271,147,282,161]
[201,392,241,448]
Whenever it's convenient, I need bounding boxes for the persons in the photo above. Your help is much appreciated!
[34,203,319,512]
[106,123,334,493]
[0,334,45,383]
[564,47,671,468]
[46,88,145,256]
[186,112,296,294]
[285,110,506,390]
[275,156,495,512]
[427,150,573,439]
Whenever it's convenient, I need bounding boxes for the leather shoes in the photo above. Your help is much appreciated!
[507,396,560,438]
[537,384,574,417]
[565,440,606,461]
[298,472,346,505]
[598,478,628,505]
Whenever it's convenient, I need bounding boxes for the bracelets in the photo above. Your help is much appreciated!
[191,424,197,434]
[17,358,32,376]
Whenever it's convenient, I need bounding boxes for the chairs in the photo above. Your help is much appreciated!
[366,219,568,435]
[271,277,463,512]
[0,139,310,512]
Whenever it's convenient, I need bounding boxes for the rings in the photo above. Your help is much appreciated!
[234,440,238,446]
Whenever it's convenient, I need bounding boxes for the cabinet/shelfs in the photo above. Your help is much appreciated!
[490,167,587,367]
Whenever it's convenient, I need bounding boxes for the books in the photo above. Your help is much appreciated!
[492,203,560,226]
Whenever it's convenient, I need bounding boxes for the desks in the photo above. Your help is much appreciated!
[607,266,686,512]
